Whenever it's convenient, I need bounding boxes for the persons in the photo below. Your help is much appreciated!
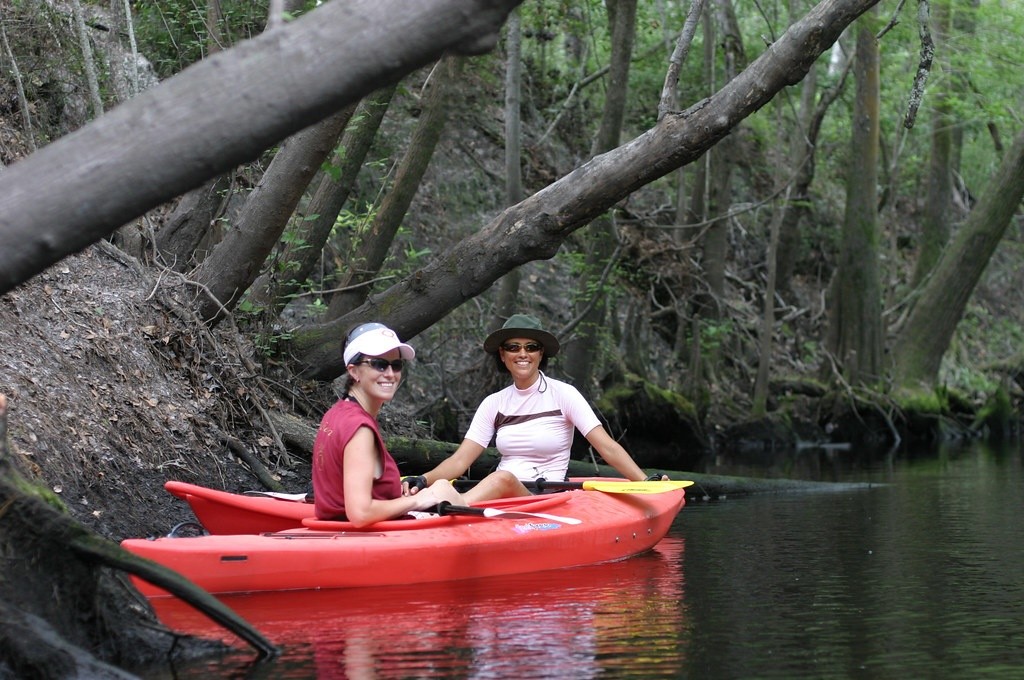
[401,314,671,504]
[312,322,465,528]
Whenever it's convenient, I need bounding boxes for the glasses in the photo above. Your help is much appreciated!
[349,358,404,372]
[500,342,544,353]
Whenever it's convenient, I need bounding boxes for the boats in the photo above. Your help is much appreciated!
[120,489,685,598]
[163,478,686,535]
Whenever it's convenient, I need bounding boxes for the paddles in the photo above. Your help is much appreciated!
[401,475,695,495]
[245,490,582,525]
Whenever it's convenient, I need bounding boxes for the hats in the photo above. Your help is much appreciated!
[342,322,415,367]
[483,314,560,357]
